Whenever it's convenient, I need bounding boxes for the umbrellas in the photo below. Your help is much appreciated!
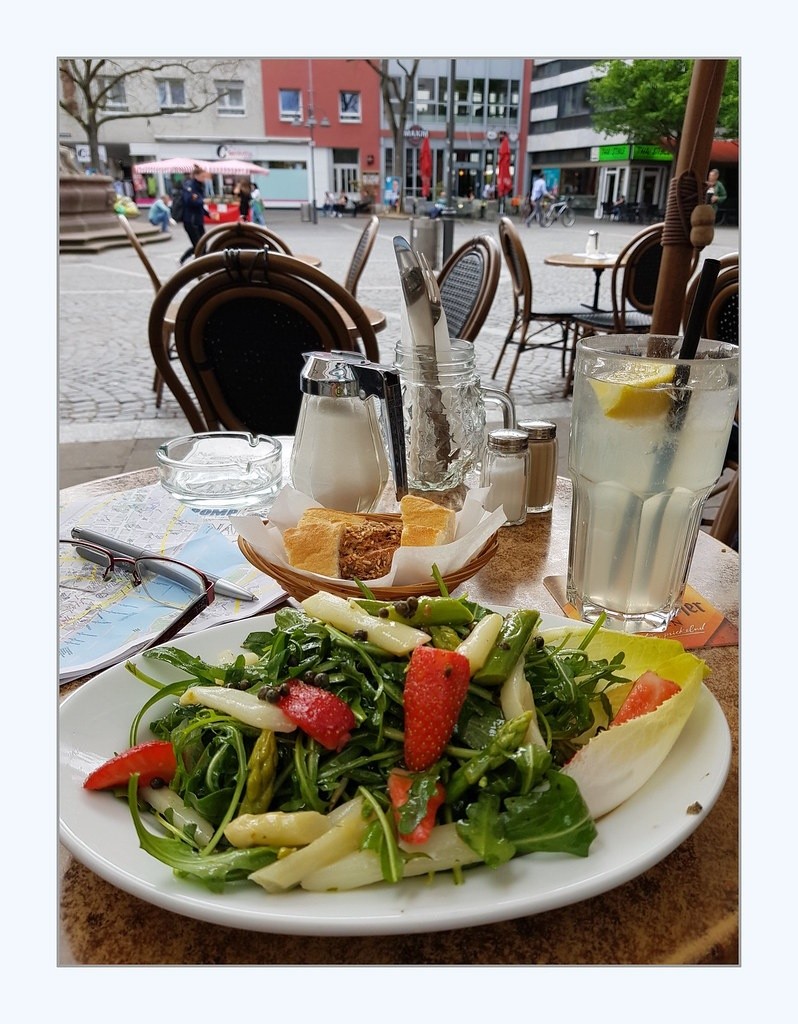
[135,156,270,198]
[420,138,432,216]
[496,136,513,214]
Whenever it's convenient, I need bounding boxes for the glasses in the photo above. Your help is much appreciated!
[58,539,214,650]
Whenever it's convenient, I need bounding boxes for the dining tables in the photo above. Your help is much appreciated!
[57,462,741,965]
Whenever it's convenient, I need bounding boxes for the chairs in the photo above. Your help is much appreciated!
[119,210,740,543]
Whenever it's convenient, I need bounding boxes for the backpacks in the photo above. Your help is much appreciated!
[172,189,184,223]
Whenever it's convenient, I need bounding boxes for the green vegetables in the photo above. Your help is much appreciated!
[300,629,713,890]
[115,563,630,887]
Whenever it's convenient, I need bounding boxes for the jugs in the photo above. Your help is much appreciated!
[289,350,408,514]
[585,229,600,255]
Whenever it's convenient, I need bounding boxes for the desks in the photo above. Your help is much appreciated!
[296,256,321,268]
[334,303,386,344]
[545,251,631,310]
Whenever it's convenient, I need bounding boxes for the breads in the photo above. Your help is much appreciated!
[281,494,457,581]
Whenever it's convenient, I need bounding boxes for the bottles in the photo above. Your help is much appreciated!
[517,420,558,513]
[478,429,531,527]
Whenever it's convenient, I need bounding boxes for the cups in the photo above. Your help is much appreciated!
[564,334,739,634]
[391,338,516,491]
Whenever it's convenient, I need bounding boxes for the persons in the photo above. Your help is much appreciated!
[179,164,219,266]
[234,177,267,227]
[323,192,355,218]
[85,157,127,197]
[611,195,646,224]
[705,169,727,213]
[149,193,176,234]
[390,179,400,206]
[430,182,496,218]
[526,173,556,227]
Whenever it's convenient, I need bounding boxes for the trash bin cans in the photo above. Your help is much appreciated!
[410,215,454,272]
[300,203,312,222]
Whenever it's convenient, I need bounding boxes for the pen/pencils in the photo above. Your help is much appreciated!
[70,528,260,602]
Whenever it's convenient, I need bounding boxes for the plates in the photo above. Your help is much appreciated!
[58,606,731,937]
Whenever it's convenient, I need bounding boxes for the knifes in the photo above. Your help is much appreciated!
[392,235,449,466]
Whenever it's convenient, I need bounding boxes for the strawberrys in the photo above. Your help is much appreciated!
[389,768,446,842]
[274,678,355,749]
[83,741,178,788]
[403,644,471,771]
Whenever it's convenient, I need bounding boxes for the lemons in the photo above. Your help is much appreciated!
[587,364,677,417]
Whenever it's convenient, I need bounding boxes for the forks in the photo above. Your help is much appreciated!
[413,251,441,327]
